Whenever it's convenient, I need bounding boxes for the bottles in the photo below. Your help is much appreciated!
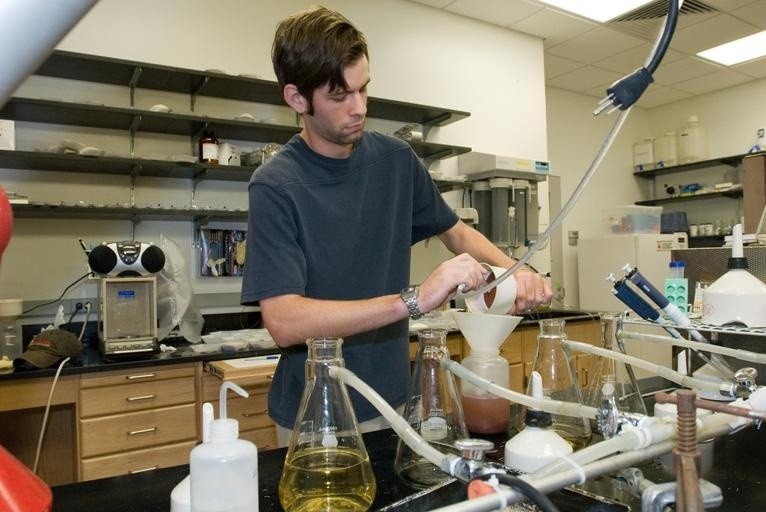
[690,215,738,237]
[276,334,379,511]
[166,378,264,512]
[391,327,472,491]
[457,348,512,433]
[199,129,220,165]
[517,317,595,439]
[502,369,574,475]
[587,309,650,434]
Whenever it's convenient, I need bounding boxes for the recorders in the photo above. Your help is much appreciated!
[89,241,166,277]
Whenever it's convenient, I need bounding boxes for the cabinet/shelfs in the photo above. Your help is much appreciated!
[633,151,766,247]
[410,320,601,405]
[0,355,280,487]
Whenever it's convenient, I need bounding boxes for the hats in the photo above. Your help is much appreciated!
[13,329,84,369]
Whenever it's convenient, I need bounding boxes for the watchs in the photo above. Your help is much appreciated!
[398,285,422,321]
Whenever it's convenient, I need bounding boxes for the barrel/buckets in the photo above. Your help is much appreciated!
[654,128,678,167]
[678,114,702,163]
[634,137,654,171]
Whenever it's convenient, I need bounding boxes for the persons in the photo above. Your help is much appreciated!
[239,3,557,448]
